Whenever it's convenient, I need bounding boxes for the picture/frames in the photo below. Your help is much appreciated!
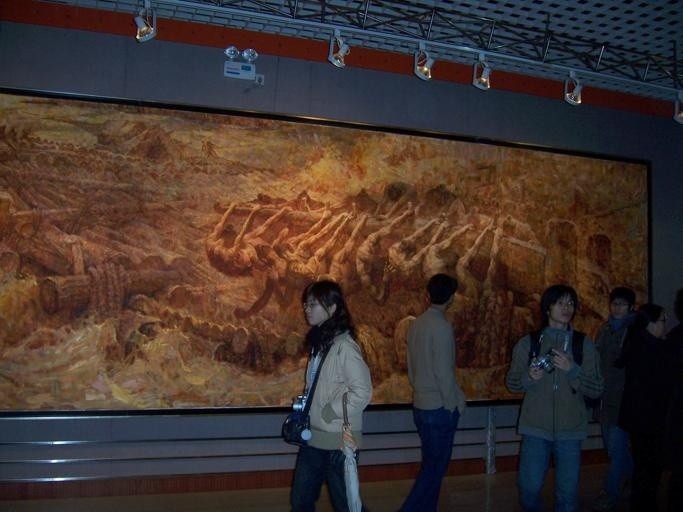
[1,86,653,420]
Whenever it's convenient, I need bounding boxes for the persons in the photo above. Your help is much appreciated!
[504,282,604,511]
[396,273,466,511]
[289,279,373,512]
[592,288,638,512]
[661,287,683,511]
[617,302,666,511]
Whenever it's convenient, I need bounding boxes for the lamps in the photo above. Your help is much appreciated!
[223,45,259,80]
[564,71,584,106]
[328,29,350,69]
[472,51,491,90]
[132,0,157,43]
[413,41,435,82]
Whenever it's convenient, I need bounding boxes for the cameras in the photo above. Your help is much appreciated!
[535,354,554,373]
[292,394,306,412]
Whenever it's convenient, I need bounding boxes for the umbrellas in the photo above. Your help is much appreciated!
[340,392,363,512]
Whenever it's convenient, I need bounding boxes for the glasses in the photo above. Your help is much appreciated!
[655,317,668,323]
[302,301,326,310]
[610,300,632,308]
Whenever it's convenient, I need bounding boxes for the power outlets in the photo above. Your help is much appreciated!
[256,73,265,86]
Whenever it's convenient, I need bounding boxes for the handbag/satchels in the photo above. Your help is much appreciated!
[281,411,310,447]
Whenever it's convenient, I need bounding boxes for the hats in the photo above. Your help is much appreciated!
[428,273,458,297]
